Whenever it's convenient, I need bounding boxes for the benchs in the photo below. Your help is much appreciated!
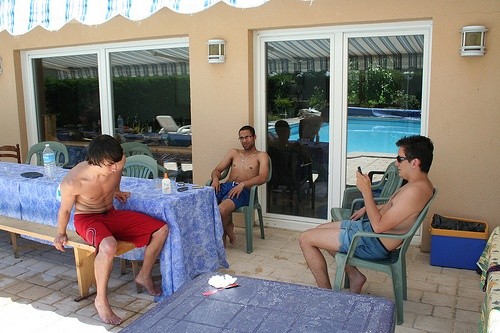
[0,216,134,297]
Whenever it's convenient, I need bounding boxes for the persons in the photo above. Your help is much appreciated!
[54,134,169,325]
[298,134,434,295]
[268,120,313,164]
[210,126,270,248]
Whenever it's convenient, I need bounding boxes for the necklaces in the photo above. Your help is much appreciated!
[242,159,244,162]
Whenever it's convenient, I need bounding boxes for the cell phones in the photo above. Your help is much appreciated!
[357,166,362,175]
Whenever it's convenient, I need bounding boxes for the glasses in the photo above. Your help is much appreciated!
[238,136,254,140]
[396,155,419,162]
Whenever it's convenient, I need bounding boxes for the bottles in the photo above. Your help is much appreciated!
[117,115,124,128]
[43,144,56,179]
[161,173,172,194]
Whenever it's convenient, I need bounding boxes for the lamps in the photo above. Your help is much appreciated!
[460,24,488,56]
[207,38,225,64]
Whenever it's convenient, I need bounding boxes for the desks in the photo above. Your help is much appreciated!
[289,141,331,180]
[474,226,500,333]
[57,133,192,170]
[0,160,229,275]
[112,272,397,333]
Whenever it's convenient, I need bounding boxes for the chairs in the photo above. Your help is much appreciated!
[156,113,193,137]
[0,141,272,256]
[271,148,317,213]
[327,161,437,325]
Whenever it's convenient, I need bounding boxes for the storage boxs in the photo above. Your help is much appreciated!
[428,211,490,271]
